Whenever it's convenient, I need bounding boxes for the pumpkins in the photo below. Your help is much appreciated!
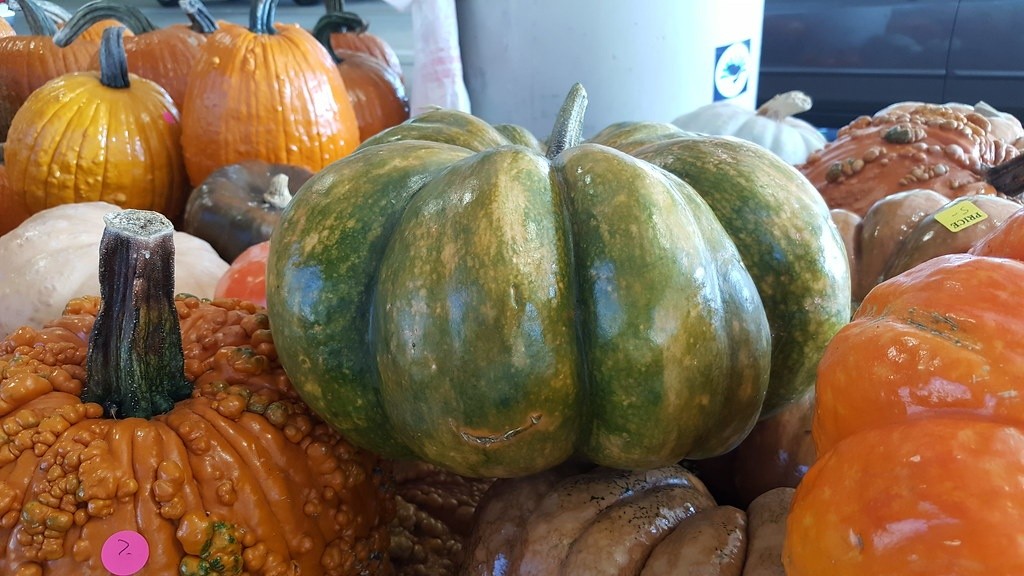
[0,0,1024,576]
